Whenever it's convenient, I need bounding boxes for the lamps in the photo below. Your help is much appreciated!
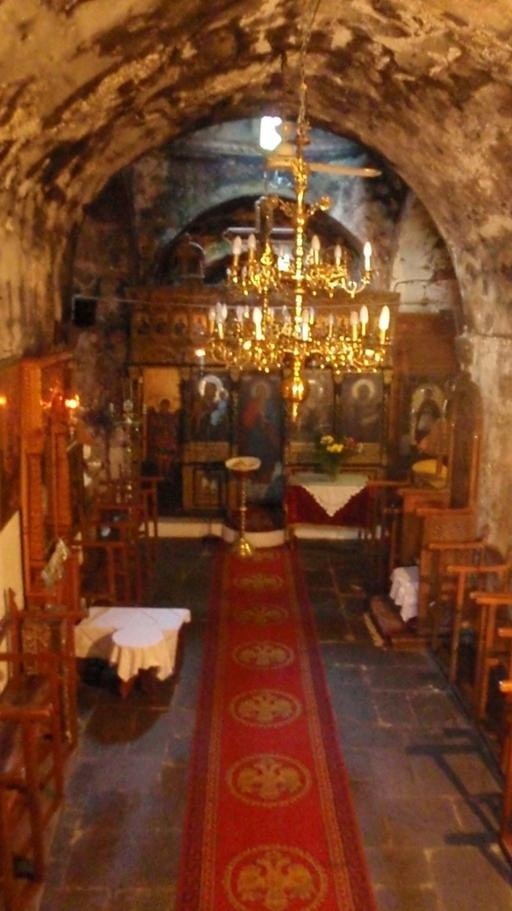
[195,0,391,431]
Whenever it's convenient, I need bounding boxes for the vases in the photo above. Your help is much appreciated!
[328,460,339,481]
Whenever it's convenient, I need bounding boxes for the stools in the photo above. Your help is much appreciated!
[110,626,165,700]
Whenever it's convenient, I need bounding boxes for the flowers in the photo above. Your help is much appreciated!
[316,434,365,460]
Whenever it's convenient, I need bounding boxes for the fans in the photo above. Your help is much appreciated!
[197,27,385,178]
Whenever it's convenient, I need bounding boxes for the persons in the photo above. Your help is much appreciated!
[150,380,441,479]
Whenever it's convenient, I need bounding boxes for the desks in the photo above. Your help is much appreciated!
[286,471,377,537]
[62,607,193,679]
